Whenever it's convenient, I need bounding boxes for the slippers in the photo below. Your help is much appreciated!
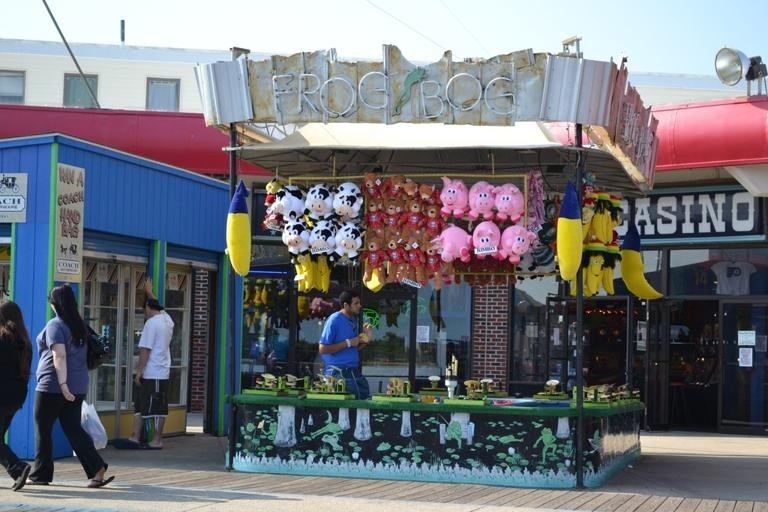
[88,476,114,488]
[112,439,162,449]
[25,477,48,485]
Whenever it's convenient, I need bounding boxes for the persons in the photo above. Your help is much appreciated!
[25,285,116,487]
[127,276,177,451]
[318,289,374,394]
[1,298,32,491]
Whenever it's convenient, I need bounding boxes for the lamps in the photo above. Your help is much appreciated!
[714,47,768,96]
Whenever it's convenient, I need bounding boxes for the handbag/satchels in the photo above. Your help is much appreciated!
[85,324,112,370]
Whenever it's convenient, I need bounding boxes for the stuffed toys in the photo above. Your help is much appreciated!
[262,176,558,292]
[223,179,251,276]
[429,289,446,328]
[244,278,340,328]
[556,179,664,301]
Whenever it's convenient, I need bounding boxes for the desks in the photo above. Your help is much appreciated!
[224,392,644,489]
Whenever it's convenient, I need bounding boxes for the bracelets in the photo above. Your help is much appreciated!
[346,338,351,349]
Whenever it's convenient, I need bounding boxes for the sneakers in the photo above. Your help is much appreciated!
[12,463,31,491]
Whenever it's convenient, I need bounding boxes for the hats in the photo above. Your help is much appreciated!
[143,299,164,310]
